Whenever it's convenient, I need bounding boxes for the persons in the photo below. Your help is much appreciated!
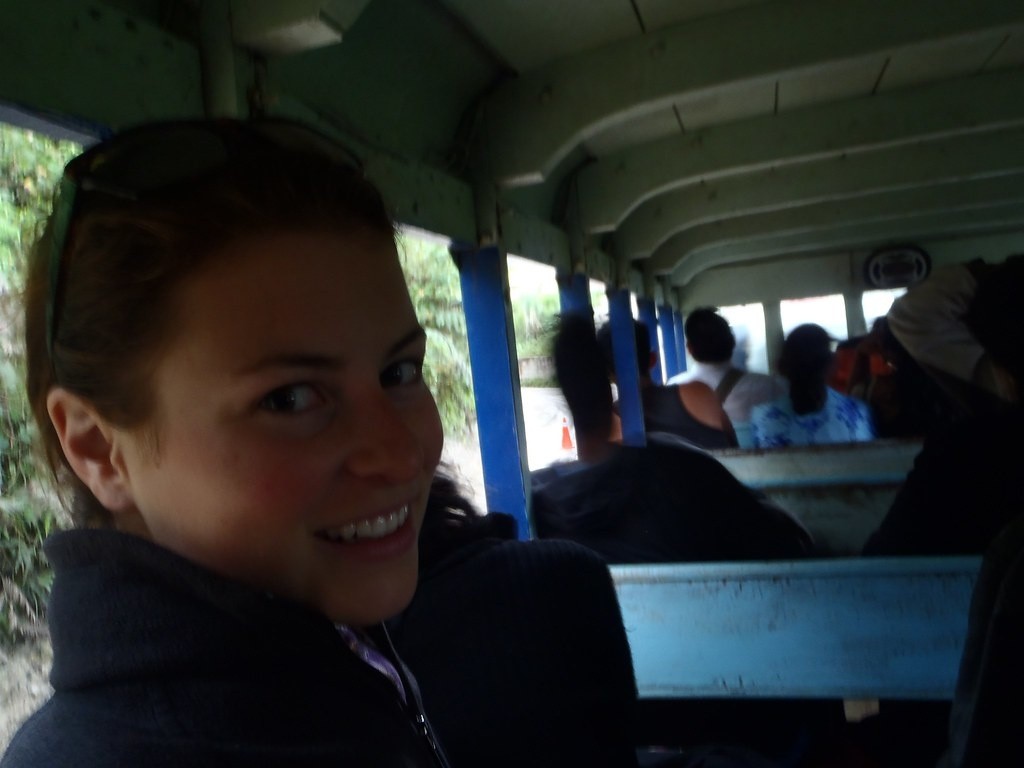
[524,314,829,768]
[1,108,661,767]
[663,308,785,444]
[840,254,1022,768]
[838,229,1005,457]
[736,326,878,450]
[597,313,738,454]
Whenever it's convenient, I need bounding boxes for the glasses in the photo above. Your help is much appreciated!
[41,114,368,385]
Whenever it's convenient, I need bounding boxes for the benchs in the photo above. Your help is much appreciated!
[709,436,923,555]
[609,556,981,719]
